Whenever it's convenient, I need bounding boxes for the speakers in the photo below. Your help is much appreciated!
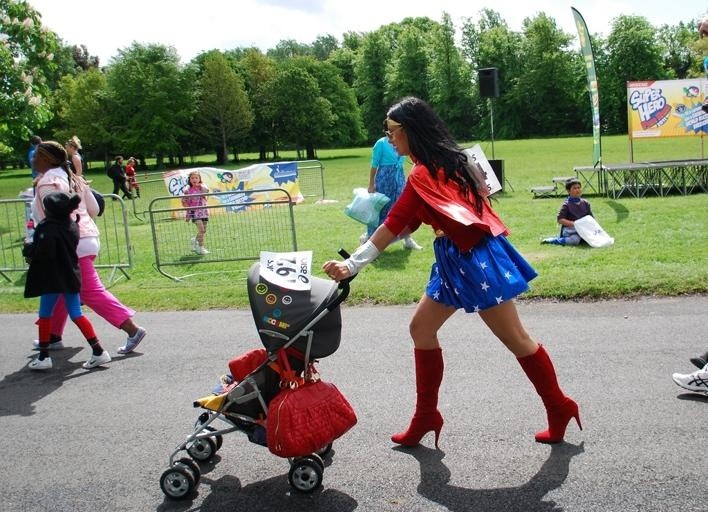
[488,160,505,192]
[478,67,500,97]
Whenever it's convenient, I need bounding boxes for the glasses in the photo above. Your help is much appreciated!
[382,127,403,139]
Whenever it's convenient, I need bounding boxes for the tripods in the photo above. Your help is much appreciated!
[488,97,515,193]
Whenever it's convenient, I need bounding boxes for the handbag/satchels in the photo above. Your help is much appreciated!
[267,379,358,457]
[343,188,391,228]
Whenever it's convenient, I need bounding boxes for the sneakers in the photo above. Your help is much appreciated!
[403,239,424,251]
[191,237,208,255]
[28,326,147,370]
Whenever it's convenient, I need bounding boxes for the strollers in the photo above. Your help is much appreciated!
[158,248,359,500]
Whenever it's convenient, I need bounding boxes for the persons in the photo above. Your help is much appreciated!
[359,118,426,250]
[181,171,210,255]
[323,100,583,444]
[22,134,147,372]
[540,179,594,247]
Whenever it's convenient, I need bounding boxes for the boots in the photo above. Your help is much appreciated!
[516,342,583,442]
[391,347,444,446]
